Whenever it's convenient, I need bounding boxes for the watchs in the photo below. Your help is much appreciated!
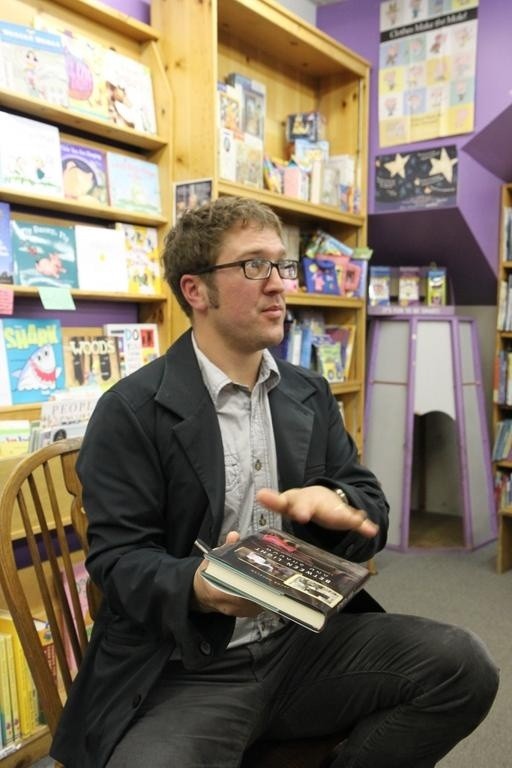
[332,486,351,508]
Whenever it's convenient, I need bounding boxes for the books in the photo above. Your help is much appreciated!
[1,202,163,295]
[6,318,168,405]
[1,386,108,463]
[3,18,158,140]
[284,307,359,382]
[491,184,512,510]
[283,224,359,299]
[367,266,447,304]
[204,524,373,633]
[2,558,95,743]
[219,73,361,212]
[2,112,161,218]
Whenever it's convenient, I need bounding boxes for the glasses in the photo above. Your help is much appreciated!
[186,258,300,280]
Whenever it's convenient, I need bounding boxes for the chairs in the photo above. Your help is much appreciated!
[1,435,104,740]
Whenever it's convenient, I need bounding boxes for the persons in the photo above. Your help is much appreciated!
[50,197,500,768]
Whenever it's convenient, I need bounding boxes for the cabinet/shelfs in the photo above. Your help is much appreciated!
[367,312,497,554]
[493,184,512,572]
[147,0,377,579]
[1,0,176,767]
